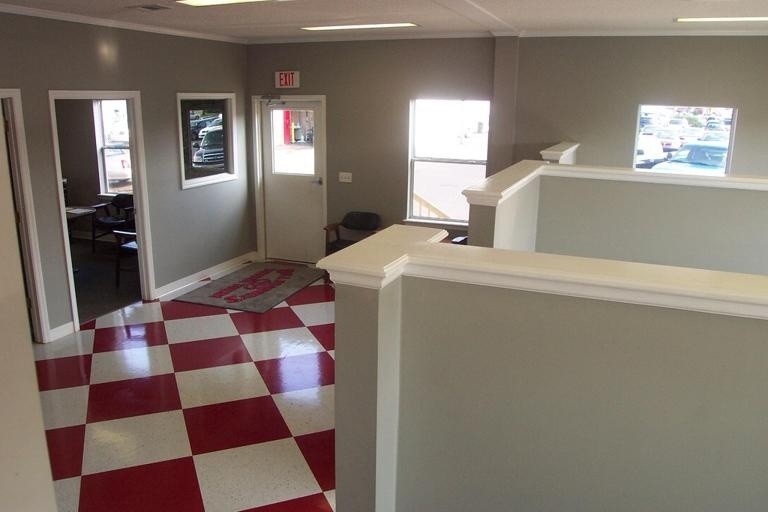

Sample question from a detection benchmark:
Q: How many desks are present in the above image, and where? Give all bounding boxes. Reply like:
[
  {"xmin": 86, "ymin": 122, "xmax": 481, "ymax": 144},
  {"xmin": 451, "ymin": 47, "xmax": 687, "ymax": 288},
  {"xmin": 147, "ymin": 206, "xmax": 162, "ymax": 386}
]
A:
[{"xmin": 66, "ymin": 204, "xmax": 96, "ymax": 255}]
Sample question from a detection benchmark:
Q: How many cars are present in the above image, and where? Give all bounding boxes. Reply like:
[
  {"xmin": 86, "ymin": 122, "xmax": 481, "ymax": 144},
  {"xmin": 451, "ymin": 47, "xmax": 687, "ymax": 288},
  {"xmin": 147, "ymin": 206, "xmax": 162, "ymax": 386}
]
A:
[
  {"xmin": 636, "ymin": 106, "xmax": 732, "ymax": 175},
  {"xmin": 190, "ymin": 114, "xmax": 224, "ymax": 170}
]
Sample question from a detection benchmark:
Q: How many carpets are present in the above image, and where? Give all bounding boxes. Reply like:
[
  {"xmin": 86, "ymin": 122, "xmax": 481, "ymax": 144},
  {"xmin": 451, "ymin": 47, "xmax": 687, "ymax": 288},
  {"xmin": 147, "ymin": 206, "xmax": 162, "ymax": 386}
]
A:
[{"xmin": 172, "ymin": 261, "xmax": 324, "ymax": 314}]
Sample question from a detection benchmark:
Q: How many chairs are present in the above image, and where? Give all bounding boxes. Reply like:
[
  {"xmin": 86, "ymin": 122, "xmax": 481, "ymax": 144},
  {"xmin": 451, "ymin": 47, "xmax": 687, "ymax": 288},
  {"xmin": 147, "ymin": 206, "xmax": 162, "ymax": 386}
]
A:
[
  {"xmin": 93, "ymin": 192, "xmax": 138, "ymax": 287},
  {"xmin": 322, "ymin": 212, "xmax": 382, "ymax": 286}
]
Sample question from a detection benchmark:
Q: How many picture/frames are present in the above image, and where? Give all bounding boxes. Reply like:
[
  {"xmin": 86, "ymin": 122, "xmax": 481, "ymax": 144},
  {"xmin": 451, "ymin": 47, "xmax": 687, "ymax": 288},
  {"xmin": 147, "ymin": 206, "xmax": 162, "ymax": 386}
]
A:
[{"xmin": 175, "ymin": 91, "xmax": 239, "ymax": 191}]
